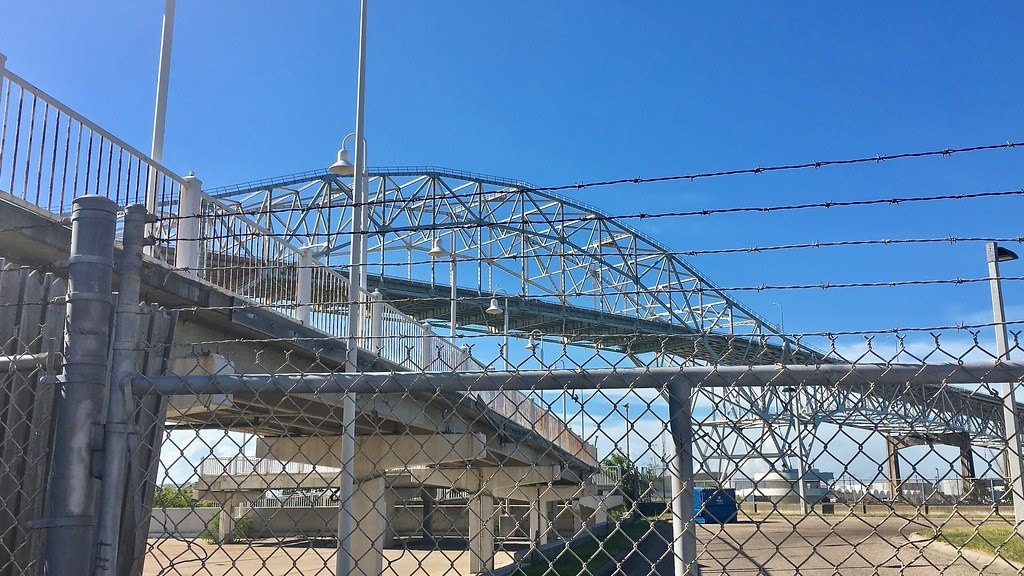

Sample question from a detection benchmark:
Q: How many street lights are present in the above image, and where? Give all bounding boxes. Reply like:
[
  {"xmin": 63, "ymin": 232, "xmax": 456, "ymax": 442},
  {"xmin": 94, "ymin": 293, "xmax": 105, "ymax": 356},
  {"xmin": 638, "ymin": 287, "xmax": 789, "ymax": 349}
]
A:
[
  {"xmin": 485, "ymin": 288, "xmax": 510, "ymax": 415},
  {"xmin": 327, "ymin": 132, "xmax": 367, "ymax": 348},
  {"xmin": 552, "ymin": 359, "xmax": 567, "ymax": 424},
  {"xmin": 427, "ymin": 226, "xmax": 456, "ymax": 373},
  {"xmin": 525, "ymin": 328, "xmax": 544, "ymax": 408},
  {"xmin": 781, "ymin": 387, "xmax": 807, "ymax": 515},
  {"xmin": 985, "ymin": 240, "xmax": 1024, "ymax": 540},
  {"xmin": 773, "ymin": 302, "xmax": 783, "ymax": 332},
  {"xmin": 621, "ymin": 402, "xmax": 629, "ymax": 459}
]
